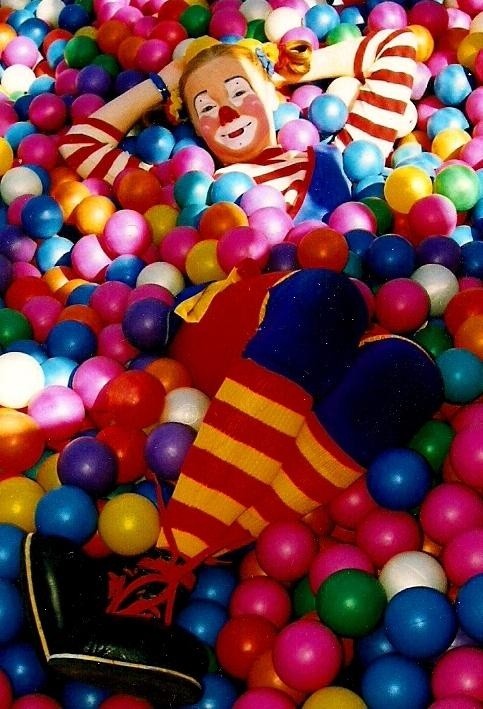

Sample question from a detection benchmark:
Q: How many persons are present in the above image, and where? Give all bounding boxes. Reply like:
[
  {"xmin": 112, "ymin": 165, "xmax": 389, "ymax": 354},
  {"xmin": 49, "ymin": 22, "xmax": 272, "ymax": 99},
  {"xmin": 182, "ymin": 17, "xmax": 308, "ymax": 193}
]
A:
[{"xmin": 22, "ymin": 26, "xmax": 446, "ymax": 707}]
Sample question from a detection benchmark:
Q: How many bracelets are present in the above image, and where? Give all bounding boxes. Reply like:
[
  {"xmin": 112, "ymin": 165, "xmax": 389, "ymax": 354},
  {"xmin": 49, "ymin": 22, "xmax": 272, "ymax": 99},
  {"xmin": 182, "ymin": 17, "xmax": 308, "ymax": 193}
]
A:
[{"xmin": 149, "ymin": 72, "xmax": 171, "ymax": 104}]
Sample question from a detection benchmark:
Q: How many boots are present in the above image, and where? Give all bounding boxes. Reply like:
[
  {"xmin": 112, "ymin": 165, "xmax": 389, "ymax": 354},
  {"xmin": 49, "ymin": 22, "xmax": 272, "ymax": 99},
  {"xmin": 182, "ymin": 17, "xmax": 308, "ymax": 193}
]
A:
[{"xmin": 25, "ymin": 532, "xmax": 208, "ymax": 703}]
